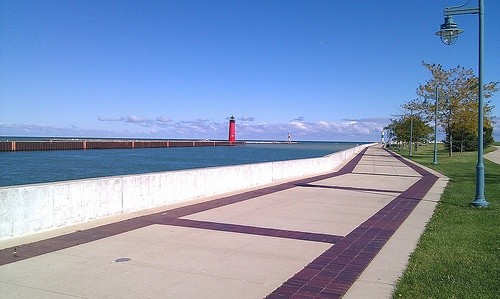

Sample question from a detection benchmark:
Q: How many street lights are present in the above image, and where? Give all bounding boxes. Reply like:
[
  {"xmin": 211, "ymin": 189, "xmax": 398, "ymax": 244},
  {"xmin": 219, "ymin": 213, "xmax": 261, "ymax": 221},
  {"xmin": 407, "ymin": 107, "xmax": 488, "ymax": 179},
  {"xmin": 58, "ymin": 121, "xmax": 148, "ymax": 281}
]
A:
[
  {"xmin": 435, "ymin": 0, "xmax": 492, "ymax": 209},
  {"xmin": 423, "ymin": 84, "xmax": 441, "ymax": 164}
]
